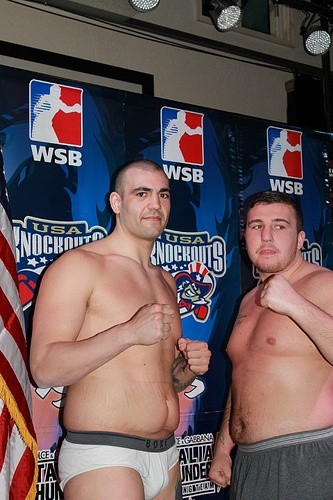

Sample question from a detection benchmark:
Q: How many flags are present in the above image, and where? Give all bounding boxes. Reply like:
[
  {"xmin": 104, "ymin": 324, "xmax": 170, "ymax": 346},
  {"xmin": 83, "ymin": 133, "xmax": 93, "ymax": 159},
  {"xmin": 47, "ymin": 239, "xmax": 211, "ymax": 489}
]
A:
[{"xmin": 0, "ymin": 145, "xmax": 39, "ymax": 500}]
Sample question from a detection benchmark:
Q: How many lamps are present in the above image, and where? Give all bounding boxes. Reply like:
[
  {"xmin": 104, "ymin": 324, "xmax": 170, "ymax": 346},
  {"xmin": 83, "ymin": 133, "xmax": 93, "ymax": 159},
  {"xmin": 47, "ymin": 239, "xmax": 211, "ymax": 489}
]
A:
[
  {"xmin": 208, "ymin": 0, "xmax": 242, "ymax": 32},
  {"xmin": 304, "ymin": 26, "xmax": 332, "ymax": 55},
  {"xmin": 128, "ymin": 0, "xmax": 161, "ymax": 12}
]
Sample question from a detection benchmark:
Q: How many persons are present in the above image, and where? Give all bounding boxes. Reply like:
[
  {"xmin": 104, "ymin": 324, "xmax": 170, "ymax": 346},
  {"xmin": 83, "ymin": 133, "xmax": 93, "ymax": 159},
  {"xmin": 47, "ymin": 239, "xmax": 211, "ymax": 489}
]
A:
[
  {"xmin": 29, "ymin": 157, "xmax": 211, "ymax": 500},
  {"xmin": 209, "ymin": 191, "xmax": 333, "ymax": 500}
]
